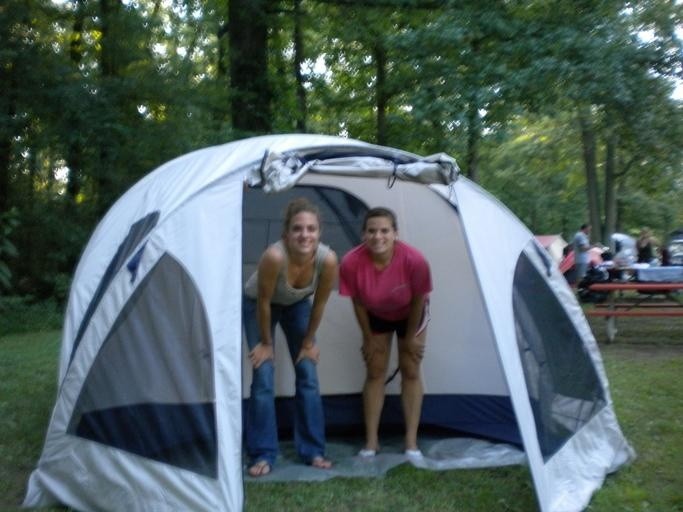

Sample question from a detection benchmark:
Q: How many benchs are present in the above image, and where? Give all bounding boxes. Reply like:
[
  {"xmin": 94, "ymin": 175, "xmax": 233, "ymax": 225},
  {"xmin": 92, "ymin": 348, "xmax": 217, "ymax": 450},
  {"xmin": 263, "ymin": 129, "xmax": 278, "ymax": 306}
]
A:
[{"xmin": 586, "ymin": 261, "xmax": 682, "ymax": 343}]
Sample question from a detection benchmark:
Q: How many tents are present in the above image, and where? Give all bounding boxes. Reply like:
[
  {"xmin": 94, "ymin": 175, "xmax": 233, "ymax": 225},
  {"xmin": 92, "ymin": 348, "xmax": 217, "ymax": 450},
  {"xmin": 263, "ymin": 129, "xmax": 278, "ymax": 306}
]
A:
[{"xmin": 22, "ymin": 134, "xmax": 637, "ymax": 512}]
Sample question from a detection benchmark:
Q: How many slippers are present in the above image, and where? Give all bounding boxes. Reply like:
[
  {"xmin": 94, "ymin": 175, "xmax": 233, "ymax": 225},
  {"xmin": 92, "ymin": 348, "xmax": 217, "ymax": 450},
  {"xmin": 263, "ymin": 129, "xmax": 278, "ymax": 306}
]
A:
[
  {"xmin": 355, "ymin": 448, "xmax": 381, "ymax": 459},
  {"xmin": 313, "ymin": 456, "xmax": 332, "ymax": 469},
  {"xmin": 403, "ymin": 449, "xmax": 424, "ymax": 460},
  {"xmin": 250, "ymin": 462, "xmax": 270, "ymax": 476}
]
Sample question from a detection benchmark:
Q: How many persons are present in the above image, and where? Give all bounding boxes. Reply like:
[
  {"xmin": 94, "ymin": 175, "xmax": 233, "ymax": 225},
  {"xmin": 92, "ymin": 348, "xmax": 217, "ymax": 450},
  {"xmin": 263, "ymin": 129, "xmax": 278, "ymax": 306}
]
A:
[
  {"xmin": 572, "ymin": 223, "xmax": 592, "ymax": 295},
  {"xmin": 575, "ymin": 251, "xmax": 617, "ymax": 300},
  {"xmin": 636, "ymin": 226, "xmax": 651, "ymax": 295},
  {"xmin": 242, "ymin": 197, "xmax": 338, "ymax": 476},
  {"xmin": 337, "ymin": 206, "xmax": 432, "ymax": 464},
  {"xmin": 654, "ymin": 246, "xmax": 673, "ymax": 268}
]
